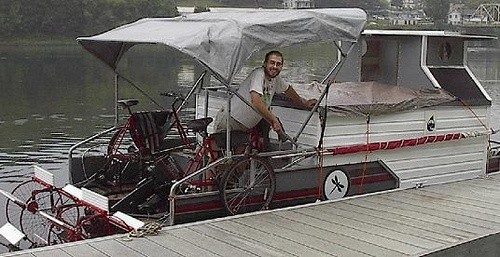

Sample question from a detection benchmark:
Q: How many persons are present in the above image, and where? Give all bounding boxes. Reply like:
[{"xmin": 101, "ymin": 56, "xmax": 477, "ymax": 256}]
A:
[{"xmin": 204, "ymin": 52, "xmax": 320, "ymax": 192}]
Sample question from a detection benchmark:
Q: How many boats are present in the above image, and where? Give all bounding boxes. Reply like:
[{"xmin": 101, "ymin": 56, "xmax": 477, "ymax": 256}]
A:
[{"xmin": 0, "ymin": 6, "xmax": 500, "ymax": 251}]
